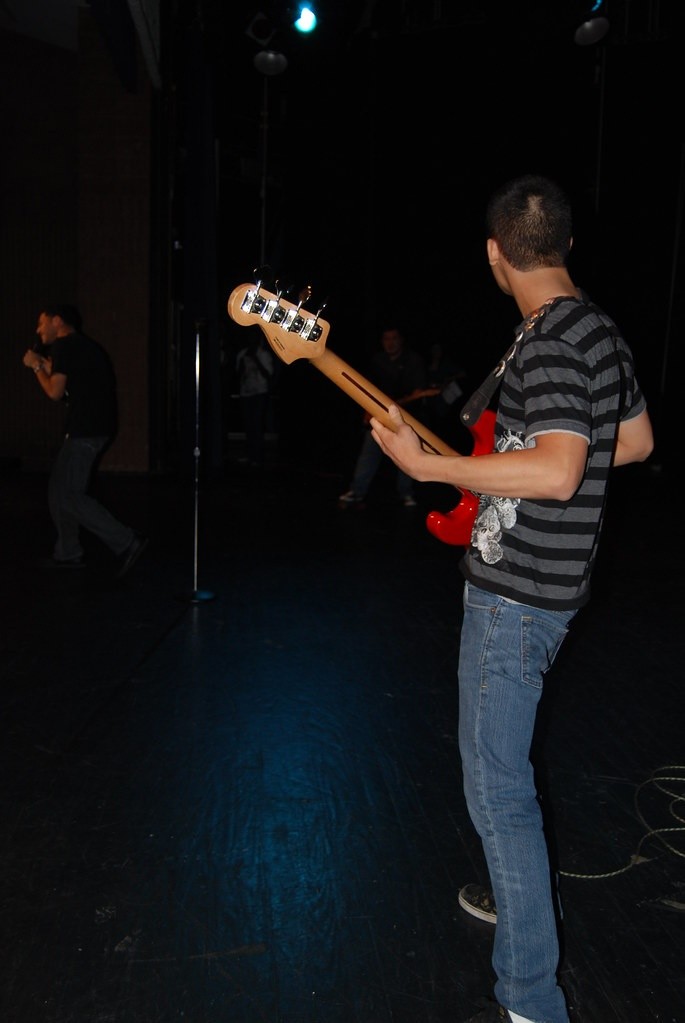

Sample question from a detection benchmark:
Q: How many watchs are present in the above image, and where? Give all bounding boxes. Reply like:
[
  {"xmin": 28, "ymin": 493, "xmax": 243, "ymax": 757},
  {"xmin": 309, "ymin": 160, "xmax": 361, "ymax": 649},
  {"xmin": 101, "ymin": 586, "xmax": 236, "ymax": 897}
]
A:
[{"xmin": 34, "ymin": 365, "xmax": 44, "ymax": 374}]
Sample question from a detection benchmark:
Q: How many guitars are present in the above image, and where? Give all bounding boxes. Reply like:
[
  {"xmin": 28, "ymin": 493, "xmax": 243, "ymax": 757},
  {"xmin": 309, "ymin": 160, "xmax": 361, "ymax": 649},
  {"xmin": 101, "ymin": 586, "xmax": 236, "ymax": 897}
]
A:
[{"xmin": 224, "ymin": 277, "xmax": 498, "ymax": 551}]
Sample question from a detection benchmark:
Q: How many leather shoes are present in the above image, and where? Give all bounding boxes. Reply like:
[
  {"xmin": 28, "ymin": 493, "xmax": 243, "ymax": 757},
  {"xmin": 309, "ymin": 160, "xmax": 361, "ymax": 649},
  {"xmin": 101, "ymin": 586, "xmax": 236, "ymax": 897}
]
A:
[
  {"xmin": 113, "ymin": 530, "xmax": 149, "ymax": 580},
  {"xmin": 36, "ymin": 552, "xmax": 91, "ymax": 569}
]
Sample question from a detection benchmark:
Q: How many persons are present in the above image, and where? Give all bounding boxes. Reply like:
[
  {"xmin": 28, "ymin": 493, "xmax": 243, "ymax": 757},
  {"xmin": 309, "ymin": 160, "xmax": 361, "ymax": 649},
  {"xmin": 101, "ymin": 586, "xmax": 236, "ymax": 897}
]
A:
[
  {"xmin": 236, "ymin": 329, "xmax": 273, "ymax": 465},
  {"xmin": 23, "ymin": 304, "xmax": 149, "ymax": 579},
  {"xmin": 369, "ymin": 175, "xmax": 654, "ymax": 1023},
  {"xmin": 339, "ymin": 327, "xmax": 417, "ymax": 506}
]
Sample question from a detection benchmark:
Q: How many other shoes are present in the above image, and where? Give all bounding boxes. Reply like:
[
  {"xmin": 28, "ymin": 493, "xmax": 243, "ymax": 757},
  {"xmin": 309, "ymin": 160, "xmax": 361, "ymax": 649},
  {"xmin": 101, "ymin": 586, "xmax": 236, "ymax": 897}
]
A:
[
  {"xmin": 402, "ymin": 493, "xmax": 417, "ymax": 506},
  {"xmin": 340, "ymin": 490, "xmax": 365, "ymax": 502},
  {"xmin": 457, "ymin": 883, "xmax": 496, "ymax": 924},
  {"xmin": 464, "ymin": 1006, "xmax": 511, "ymax": 1023}
]
